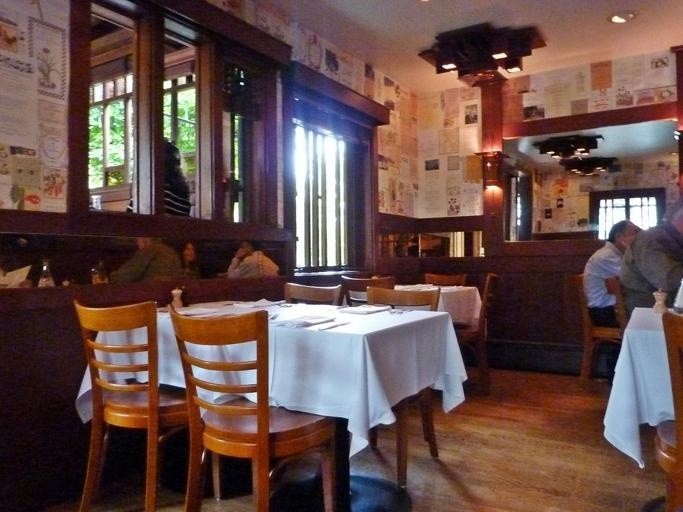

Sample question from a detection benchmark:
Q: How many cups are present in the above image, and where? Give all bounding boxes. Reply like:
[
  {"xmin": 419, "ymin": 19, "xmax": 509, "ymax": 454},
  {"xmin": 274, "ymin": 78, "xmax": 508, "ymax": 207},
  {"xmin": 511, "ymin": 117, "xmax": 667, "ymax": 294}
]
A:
[{"xmin": 90, "ymin": 266, "xmax": 105, "ymax": 284}]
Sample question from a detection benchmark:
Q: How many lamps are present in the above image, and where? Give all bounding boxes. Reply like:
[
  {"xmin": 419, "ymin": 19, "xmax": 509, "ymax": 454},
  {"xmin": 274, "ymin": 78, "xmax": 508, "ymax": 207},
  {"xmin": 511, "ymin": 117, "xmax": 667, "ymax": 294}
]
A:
[
  {"xmin": 416, "ymin": 23, "xmax": 548, "ymax": 88},
  {"xmin": 558, "ymin": 157, "xmax": 618, "ymax": 178},
  {"xmin": 472, "ymin": 150, "xmax": 510, "ymax": 190},
  {"xmin": 530, "ymin": 134, "xmax": 605, "ymax": 160}
]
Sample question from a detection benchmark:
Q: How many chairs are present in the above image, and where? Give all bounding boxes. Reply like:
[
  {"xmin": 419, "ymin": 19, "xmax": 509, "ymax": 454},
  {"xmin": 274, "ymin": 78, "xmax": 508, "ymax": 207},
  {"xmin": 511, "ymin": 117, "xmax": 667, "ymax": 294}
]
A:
[
  {"xmin": 68, "ymin": 300, "xmax": 221, "ymax": 510},
  {"xmin": 572, "ymin": 265, "xmax": 682, "ymax": 510},
  {"xmin": 330, "ymin": 269, "xmax": 507, "ymax": 405},
  {"xmin": 362, "ymin": 280, "xmax": 443, "ymax": 491},
  {"xmin": 283, "ymin": 280, "xmax": 343, "ymax": 306},
  {"xmin": 166, "ymin": 302, "xmax": 348, "ymax": 512}
]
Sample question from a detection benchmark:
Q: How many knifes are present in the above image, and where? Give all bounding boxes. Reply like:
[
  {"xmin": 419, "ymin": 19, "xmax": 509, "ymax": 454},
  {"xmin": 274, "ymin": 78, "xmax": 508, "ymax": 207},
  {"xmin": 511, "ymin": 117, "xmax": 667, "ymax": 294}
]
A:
[{"xmin": 317, "ymin": 320, "xmax": 350, "ymax": 332}]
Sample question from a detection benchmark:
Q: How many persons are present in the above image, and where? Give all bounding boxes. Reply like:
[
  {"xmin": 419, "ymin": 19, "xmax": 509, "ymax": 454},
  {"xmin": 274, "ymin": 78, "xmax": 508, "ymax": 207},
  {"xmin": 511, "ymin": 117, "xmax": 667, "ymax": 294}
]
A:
[
  {"xmin": 178, "ymin": 241, "xmax": 207, "ymax": 278},
  {"xmin": 126, "ymin": 138, "xmax": 191, "ymax": 216},
  {"xmin": 582, "ymin": 219, "xmax": 639, "ymax": 383},
  {"xmin": 107, "ymin": 237, "xmax": 184, "ymax": 282},
  {"xmin": 618, "ymin": 194, "xmax": 683, "ymax": 318},
  {"xmin": 226, "ymin": 240, "xmax": 278, "ymax": 277}
]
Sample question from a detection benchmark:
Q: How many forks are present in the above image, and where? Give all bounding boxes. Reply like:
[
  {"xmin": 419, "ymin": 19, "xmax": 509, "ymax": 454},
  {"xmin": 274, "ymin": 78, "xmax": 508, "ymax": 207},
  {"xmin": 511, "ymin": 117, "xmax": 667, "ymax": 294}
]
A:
[{"xmin": 270, "ymin": 313, "xmax": 281, "ymax": 320}]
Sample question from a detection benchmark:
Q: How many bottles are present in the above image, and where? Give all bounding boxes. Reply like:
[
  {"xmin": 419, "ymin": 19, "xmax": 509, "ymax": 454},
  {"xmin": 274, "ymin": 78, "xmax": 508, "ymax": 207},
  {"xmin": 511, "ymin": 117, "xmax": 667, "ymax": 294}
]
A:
[
  {"xmin": 170, "ymin": 287, "xmax": 183, "ymax": 310},
  {"xmin": 672, "ymin": 277, "xmax": 683, "ymax": 314},
  {"xmin": 181, "ymin": 285, "xmax": 190, "ymax": 309},
  {"xmin": 651, "ymin": 288, "xmax": 670, "ymax": 316},
  {"xmin": 37, "ymin": 259, "xmax": 56, "ymax": 288}
]
